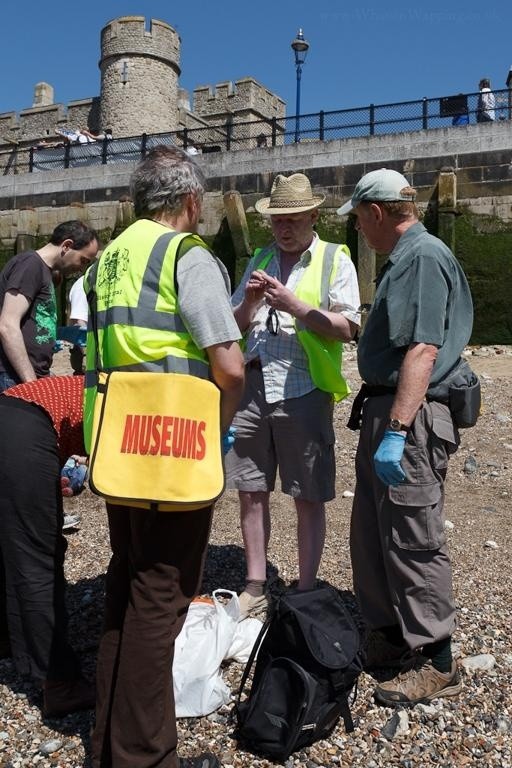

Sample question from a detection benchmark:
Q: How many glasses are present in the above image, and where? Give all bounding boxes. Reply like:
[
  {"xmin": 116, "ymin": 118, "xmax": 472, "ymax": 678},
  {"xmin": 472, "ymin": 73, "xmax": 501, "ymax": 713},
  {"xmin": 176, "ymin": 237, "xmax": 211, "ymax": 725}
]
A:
[{"xmin": 265, "ymin": 305, "xmax": 280, "ymax": 337}]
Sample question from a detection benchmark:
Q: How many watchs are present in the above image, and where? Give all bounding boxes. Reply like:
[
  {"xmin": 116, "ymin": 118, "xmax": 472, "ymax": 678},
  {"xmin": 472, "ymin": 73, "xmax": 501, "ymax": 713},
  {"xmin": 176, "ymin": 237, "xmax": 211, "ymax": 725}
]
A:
[{"xmin": 386, "ymin": 417, "xmax": 411, "ymax": 433}]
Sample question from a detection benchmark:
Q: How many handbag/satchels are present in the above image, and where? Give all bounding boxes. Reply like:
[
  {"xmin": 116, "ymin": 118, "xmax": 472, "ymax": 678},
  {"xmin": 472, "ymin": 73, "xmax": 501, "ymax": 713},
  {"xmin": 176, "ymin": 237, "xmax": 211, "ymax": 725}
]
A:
[
  {"xmin": 448, "ymin": 367, "xmax": 481, "ymax": 428},
  {"xmin": 83, "ymin": 366, "xmax": 226, "ymax": 514},
  {"xmin": 172, "ymin": 587, "xmax": 250, "ymax": 723}
]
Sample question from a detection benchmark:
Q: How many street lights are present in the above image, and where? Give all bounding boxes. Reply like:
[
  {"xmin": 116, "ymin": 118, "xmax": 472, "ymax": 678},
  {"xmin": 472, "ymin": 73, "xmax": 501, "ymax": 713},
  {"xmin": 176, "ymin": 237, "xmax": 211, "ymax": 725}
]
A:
[{"xmin": 291, "ymin": 27, "xmax": 309, "ymax": 144}]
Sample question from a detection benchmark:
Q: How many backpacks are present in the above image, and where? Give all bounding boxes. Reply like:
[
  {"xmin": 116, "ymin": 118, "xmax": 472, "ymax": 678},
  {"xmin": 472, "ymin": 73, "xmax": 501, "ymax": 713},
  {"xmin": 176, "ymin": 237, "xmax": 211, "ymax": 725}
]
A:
[{"xmin": 231, "ymin": 583, "xmax": 366, "ymax": 764}]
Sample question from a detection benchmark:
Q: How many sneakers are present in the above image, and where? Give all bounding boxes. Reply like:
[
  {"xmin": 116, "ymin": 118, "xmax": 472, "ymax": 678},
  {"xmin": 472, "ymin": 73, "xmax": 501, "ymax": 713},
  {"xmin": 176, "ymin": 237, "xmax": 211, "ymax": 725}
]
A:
[
  {"xmin": 373, "ymin": 654, "xmax": 463, "ymax": 708},
  {"xmin": 42, "ymin": 675, "xmax": 98, "ymax": 719},
  {"xmin": 176, "ymin": 752, "xmax": 222, "ymax": 768},
  {"xmin": 358, "ymin": 628, "xmax": 410, "ymax": 674},
  {"xmin": 227, "ymin": 590, "xmax": 270, "ymax": 625}
]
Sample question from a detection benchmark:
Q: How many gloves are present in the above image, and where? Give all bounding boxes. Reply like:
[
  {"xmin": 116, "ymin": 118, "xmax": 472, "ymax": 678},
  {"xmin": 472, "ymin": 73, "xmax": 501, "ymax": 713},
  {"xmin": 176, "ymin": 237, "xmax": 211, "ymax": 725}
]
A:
[
  {"xmin": 222, "ymin": 426, "xmax": 237, "ymax": 456},
  {"xmin": 373, "ymin": 426, "xmax": 409, "ymax": 486}
]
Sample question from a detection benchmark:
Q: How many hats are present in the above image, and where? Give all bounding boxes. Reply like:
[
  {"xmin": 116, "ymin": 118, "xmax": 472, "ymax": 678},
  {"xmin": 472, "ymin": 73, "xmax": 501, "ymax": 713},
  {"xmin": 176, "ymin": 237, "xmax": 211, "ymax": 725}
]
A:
[
  {"xmin": 254, "ymin": 172, "xmax": 326, "ymax": 215},
  {"xmin": 335, "ymin": 167, "xmax": 417, "ymax": 216}
]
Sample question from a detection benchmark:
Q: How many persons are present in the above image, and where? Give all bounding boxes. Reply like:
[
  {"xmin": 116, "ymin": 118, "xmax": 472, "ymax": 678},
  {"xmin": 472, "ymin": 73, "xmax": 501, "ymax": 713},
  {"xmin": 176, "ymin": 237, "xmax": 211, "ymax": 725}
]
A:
[
  {"xmin": 336, "ymin": 168, "xmax": 481, "ymax": 707},
  {"xmin": 54, "ymin": 126, "xmax": 113, "ymax": 143},
  {"xmin": 0, "ymin": 219, "xmax": 99, "ymax": 715},
  {"xmin": 83, "ymin": 144, "xmax": 246, "ymax": 768},
  {"xmin": 452, "ymin": 78, "xmax": 496, "ymax": 125},
  {"xmin": 232, "ymin": 173, "xmax": 361, "ymax": 613}
]
